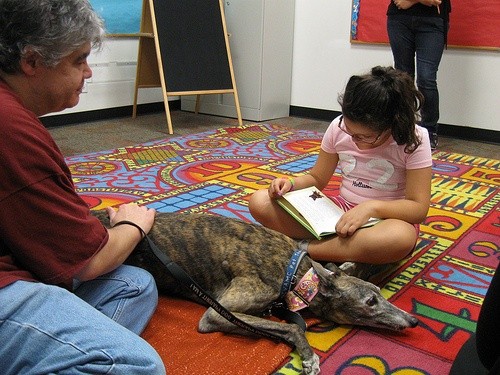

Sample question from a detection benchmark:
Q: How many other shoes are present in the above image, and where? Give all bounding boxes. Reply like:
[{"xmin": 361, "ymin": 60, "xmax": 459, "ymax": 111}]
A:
[
  {"xmin": 338, "ymin": 261, "xmax": 374, "ymax": 278},
  {"xmin": 428, "ymin": 133, "xmax": 439, "ymax": 149}
]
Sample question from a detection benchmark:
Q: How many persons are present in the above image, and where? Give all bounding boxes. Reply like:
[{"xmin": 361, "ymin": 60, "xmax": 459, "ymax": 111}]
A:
[
  {"xmin": 387, "ymin": 0, "xmax": 452, "ymax": 150},
  {"xmin": 0, "ymin": 0, "xmax": 166, "ymax": 375},
  {"xmin": 249, "ymin": 66, "xmax": 432, "ymax": 263}
]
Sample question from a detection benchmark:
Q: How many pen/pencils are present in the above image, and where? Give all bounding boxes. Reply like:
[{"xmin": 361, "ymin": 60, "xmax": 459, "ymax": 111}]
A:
[{"xmin": 437, "ymin": 5, "xmax": 442, "ymax": 15}]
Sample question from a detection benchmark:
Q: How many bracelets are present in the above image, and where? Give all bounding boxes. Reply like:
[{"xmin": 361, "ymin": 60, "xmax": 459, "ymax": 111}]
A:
[{"xmin": 287, "ymin": 177, "xmax": 294, "ymax": 191}]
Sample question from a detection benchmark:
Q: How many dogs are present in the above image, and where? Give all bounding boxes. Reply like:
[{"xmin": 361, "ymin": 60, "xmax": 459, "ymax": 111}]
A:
[{"xmin": 88, "ymin": 207, "xmax": 421, "ymax": 375}]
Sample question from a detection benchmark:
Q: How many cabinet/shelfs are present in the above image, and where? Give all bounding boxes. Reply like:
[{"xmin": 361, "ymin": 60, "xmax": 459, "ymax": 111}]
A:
[{"xmin": 181, "ymin": 0, "xmax": 297, "ymax": 122}]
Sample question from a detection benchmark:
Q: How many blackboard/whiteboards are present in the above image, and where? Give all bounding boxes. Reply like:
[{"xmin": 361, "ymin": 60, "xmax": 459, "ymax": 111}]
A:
[{"xmin": 128, "ymin": 0, "xmax": 246, "ymax": 137}]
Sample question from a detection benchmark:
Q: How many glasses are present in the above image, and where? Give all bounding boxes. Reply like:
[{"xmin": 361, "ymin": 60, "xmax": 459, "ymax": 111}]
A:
[{"xmin": 337, "ymin": 116, "xmax": 382, "ymax": 147}]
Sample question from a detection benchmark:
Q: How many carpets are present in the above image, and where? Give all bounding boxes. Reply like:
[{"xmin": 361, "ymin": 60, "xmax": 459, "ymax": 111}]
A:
[{"xmin": 65, "ymin": 123, "xmax": 499, "ymax": 375}]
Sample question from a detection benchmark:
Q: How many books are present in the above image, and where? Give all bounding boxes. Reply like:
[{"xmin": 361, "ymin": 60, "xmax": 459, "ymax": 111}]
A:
[{"xmin": 277, "ymin": 185, "xmax": 380, "ymax": 241}]
